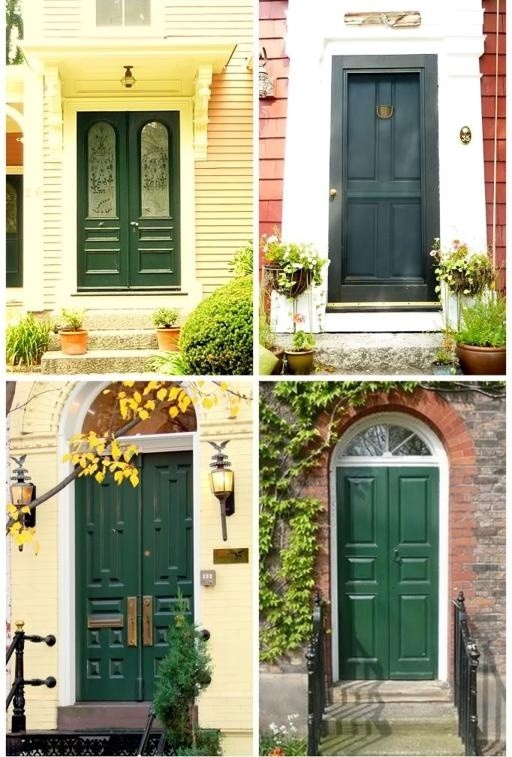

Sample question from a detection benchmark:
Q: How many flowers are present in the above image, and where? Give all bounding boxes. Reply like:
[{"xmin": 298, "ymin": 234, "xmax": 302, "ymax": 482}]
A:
[
  {"xmin": 430, "ymin": 238, "xmax": 485, "ymax": 274},
  {"xmin": 262, "ymin": 240, "xmax": 330, "ymax": 271}
]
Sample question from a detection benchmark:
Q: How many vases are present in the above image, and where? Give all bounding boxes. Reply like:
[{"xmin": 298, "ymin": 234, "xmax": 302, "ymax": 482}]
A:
[
  {"xmin": 269, "ymin": 271, "xmax": 318, "ymax": 296},
  {"xmin": 446, "ymin": 272, "xmax": 477, "ymax": 292}
]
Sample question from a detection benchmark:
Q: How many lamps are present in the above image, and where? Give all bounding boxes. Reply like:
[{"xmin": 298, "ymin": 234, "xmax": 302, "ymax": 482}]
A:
[
  {"xmin": 210, "ymin": 438, "xmax": 234, "ymax": 542},
  {"xmin": 7, "ymin": 453, "xmax": 38, "ymax": 555},
  {"xmin": 122, "ymin": 66, "xmax": 138, "ymax": 87}
]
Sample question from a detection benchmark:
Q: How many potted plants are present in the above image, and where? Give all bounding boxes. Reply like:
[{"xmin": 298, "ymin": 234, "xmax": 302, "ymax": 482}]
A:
[
  {"xmin": 286, "ymin": 330, "xmax": 318, "ymax": 370},
  {"xmin": 457, "ymin": 293, "xmax": 507, "ymax": 376},
  {"xmin": 152, "ymin": 305, "xmax": 182, "ymax": 349},
  {"xmin": 59, "ymin": 306, "xmax": 89, "ymax": 355}
]
